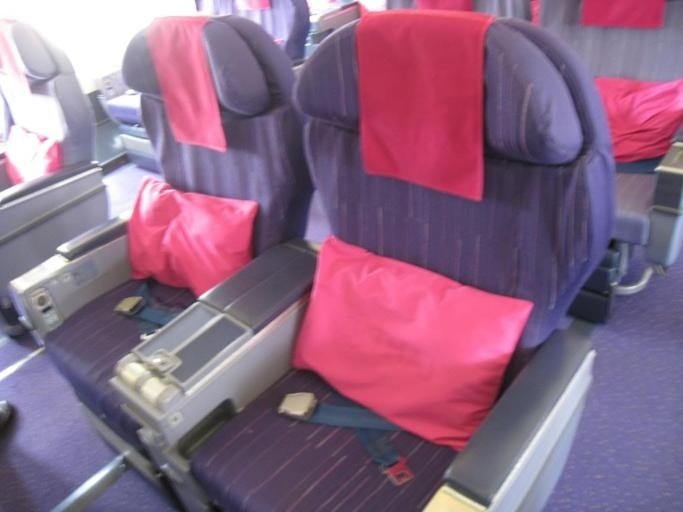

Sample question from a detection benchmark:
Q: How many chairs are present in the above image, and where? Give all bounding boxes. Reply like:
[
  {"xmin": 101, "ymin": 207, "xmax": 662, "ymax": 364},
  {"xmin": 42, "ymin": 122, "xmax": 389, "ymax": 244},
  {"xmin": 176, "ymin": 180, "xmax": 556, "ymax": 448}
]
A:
[
  {"xmin": 103, "ymin": 2, "xmax": 307, "ymax": 171},
  {"xmin": 1, "ymin": 11, "xmax": 110, "ymax": 337},
  {"xmin": 311, "ymin": 2, "xmax": 530, "ymax": 38},
  {"xmin": 510, "ymin": 1, "xmax": 682, "ymax": 293},
  {"xmin": 138, "ymin": 6, "xmax": 616, "ymax": 512},
  {"xmin": 6, "ymin": 14, "xmax": 313, "ymax": 493}
]
